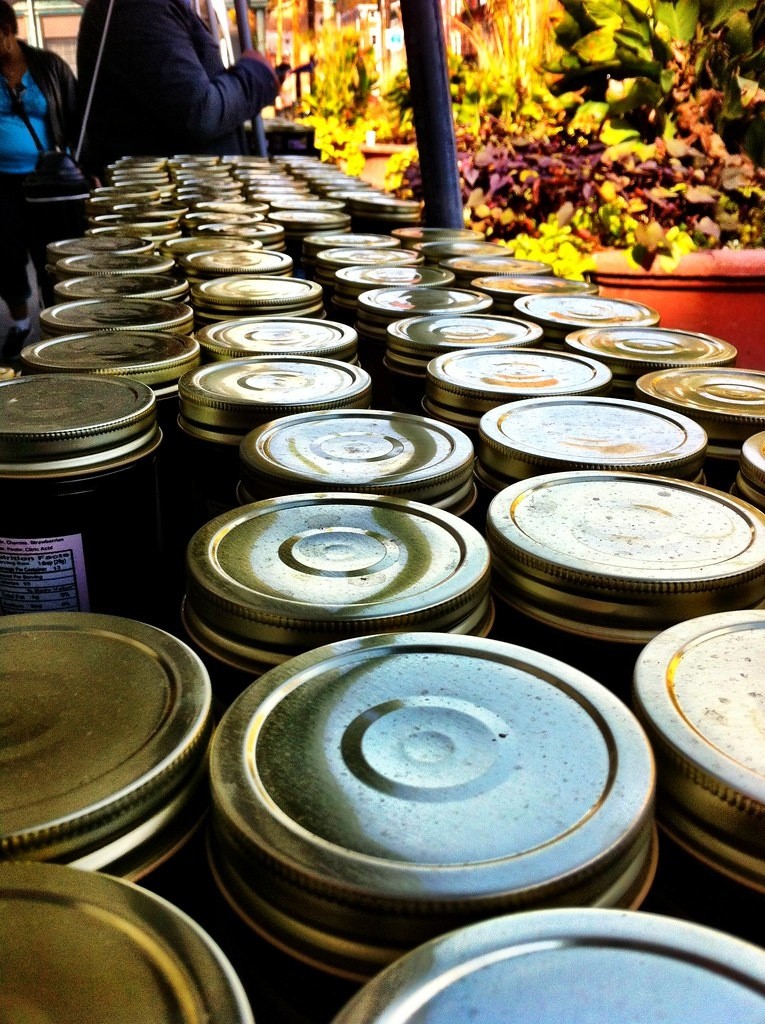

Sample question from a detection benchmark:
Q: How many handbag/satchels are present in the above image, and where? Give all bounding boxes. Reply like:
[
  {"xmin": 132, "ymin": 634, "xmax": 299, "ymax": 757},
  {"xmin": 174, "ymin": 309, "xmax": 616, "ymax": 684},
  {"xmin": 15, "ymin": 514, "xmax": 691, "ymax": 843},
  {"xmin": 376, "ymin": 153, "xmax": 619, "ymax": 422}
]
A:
[{"xmin": 24, "ymin": 153, "xmax": 91, "ymax": 232}]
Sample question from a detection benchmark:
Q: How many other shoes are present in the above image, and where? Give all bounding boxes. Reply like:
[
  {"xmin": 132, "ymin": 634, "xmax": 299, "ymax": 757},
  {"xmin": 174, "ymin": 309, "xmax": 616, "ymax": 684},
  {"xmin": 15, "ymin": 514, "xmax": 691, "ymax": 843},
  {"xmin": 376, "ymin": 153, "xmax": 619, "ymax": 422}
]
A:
[{"xmin": 0, "ymin": 325, "xmax": 34, "ymax": 362}]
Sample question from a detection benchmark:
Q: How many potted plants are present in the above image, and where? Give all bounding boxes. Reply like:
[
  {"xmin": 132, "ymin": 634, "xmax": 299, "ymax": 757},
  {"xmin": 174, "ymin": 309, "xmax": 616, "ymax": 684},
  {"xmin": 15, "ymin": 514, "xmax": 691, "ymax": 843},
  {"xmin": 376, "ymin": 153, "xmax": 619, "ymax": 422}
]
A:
[
  {"xmin": 357, "ymin": 68, "xmax": 416, "ymax": 187},
  {"xmin": 541, "ymin": 0, "xmax": 765, "ymax": 372}
]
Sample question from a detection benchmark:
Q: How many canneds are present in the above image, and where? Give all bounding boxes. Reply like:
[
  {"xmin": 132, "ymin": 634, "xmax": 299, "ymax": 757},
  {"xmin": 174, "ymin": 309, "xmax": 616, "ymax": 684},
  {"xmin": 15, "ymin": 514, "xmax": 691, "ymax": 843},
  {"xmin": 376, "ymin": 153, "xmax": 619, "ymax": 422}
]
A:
[{"xmin": 1, "ymin": 156, "xmax": 764, "ymax": 1024}]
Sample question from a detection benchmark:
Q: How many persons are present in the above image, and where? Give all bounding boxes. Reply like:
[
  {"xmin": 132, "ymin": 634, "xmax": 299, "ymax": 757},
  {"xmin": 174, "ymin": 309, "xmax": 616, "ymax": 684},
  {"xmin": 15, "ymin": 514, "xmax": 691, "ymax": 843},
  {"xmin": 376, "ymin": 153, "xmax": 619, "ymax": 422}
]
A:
[
  {"xmin": 75, "ymin": 1, "xmax": 282, "ymax": 188},
  {"xmin": 0, "ymin": 1, "xmax": 87, "ymax": 358}
]
